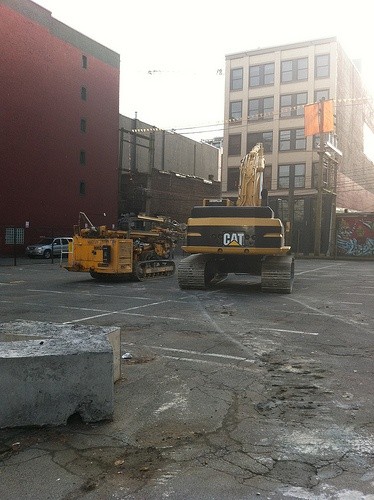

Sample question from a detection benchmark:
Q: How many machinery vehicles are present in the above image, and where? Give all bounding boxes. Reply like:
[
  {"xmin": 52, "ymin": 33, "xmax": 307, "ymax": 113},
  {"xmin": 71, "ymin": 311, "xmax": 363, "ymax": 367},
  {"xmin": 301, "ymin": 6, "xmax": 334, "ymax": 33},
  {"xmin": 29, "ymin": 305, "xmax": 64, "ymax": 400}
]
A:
[
  {"xmin": 176, "ymin": 141, "xmax": 294, "ymax": 294},
  {"xmin": 65, "ymin": 211, "xmax": 185, "ymax": 281}
]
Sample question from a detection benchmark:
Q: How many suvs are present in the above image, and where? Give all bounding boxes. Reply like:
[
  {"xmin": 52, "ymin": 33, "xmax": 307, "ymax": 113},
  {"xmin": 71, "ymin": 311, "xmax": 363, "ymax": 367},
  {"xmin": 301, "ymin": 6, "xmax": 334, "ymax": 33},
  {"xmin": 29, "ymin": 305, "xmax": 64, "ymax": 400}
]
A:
[{"xmin": 25, "ymin": 237, "xmax": 74, "ymax": 258}]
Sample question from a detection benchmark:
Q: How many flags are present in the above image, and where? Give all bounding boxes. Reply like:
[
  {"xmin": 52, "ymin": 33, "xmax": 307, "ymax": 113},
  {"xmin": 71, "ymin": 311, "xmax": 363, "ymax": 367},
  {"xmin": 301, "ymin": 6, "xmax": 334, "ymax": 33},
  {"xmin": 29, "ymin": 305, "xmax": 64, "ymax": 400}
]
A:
[{"xmin": 303, "ymin": 99, "xmax": 335, "ymax": 138}]
